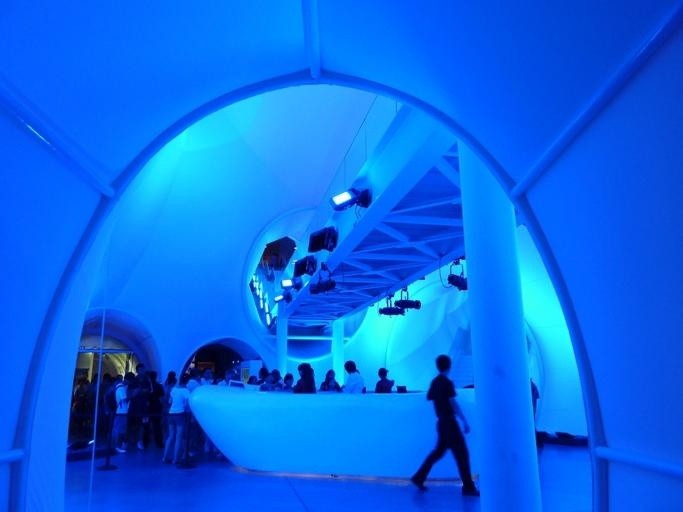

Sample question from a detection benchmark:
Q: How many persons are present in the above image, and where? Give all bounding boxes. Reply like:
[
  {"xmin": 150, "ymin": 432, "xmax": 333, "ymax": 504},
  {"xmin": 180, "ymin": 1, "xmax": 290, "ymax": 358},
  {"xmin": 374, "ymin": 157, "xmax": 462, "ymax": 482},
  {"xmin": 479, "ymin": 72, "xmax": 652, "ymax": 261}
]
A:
[
  {"xmin": 341, "ymin": 361, "xmax": 366, "ymax": 394},
  {"xmin": 291, "ymin": 363, "xmax": 316, "ymax": 393},
  {"xmin": 409, "ymin": 355, "xmax": 480, "ymax": 496},
  {"xmin": 69, "ymin": 363, "xmax": 240, "ymax": 467},
  {"xmin": 248, "ymin": 368, "xmax": 294, "ymax": 392},
  {"xmin": 318, "ymin": 370, "xmax": 341, "ymax": 393},
  {"xmin": 375, "ymin": 368, "xmax": 394, "ymax": 392}
]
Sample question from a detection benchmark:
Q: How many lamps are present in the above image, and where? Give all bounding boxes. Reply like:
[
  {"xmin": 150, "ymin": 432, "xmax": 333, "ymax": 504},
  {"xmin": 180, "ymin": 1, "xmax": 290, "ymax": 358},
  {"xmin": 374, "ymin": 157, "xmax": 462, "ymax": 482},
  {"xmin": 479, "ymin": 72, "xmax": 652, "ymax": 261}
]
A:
[
  {"xmin": 380, "ymin": 253, "xmax": 468, "ymax": 318},
  {"xmin": 273, "ymin": 184, "xmax": 372, "ymax": 304}
]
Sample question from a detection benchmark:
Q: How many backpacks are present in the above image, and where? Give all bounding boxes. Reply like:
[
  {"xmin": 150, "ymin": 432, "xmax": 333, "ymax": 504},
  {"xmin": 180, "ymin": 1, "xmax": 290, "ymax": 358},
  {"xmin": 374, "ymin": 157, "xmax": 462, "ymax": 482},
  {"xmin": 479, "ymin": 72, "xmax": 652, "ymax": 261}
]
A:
[{"xmin": 103, "ymin": 383, "xmax": 125, "ymax": 415}]
